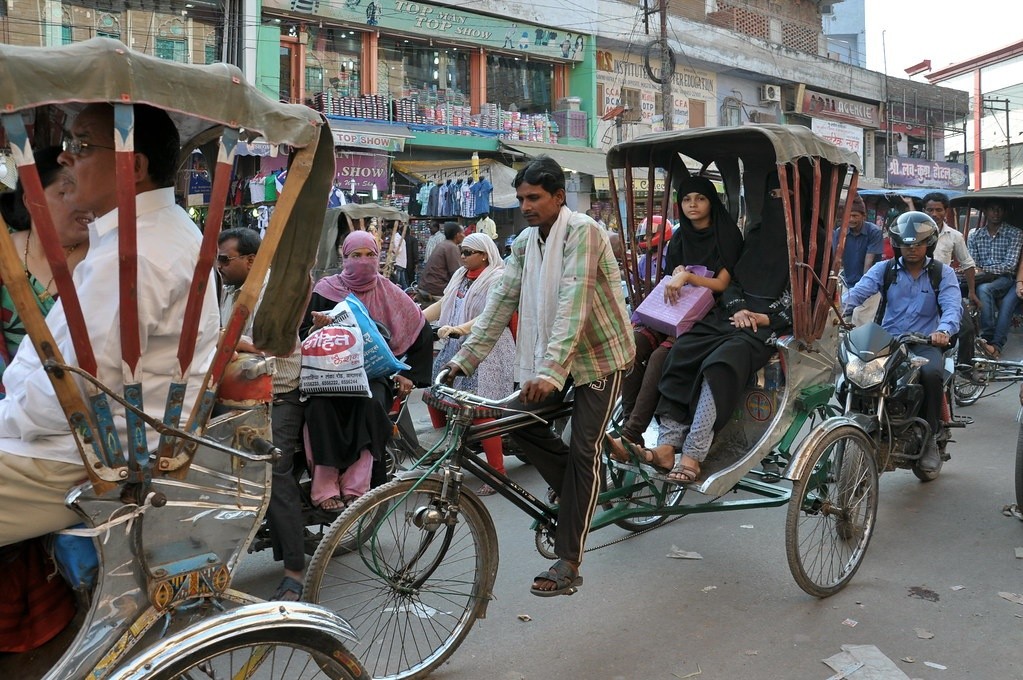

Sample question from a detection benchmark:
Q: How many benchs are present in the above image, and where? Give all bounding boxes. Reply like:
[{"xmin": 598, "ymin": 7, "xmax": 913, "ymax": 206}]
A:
[
  {"xmin": 749, "ymin": 303, "xmax": 844, "ymax": 389},
  {"xmin": 52, "ymin": 355, "xmax": 279, "ymax": 590}
]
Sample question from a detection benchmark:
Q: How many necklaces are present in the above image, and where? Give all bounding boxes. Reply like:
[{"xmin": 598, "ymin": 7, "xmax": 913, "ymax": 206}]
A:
[{"xmin": 25, "ymin": 227, "xmax": 80, "ymax": 302}]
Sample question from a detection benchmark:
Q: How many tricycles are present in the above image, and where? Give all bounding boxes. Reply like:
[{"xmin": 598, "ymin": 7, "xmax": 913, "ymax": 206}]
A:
[
  {"xmin": 950, "ymin": 184, "xmax": 1023, "ymax": 407},
  {"xmin": 295, "ymin": 120, "xmax": 883, "ymax": 680},
  {"xmin": 0, "ymin": 32, "xmax": 377, "ymax": 680}
]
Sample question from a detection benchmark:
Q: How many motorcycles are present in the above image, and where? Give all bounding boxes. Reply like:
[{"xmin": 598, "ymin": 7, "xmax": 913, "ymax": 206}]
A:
[{"xmin": 833, "ymin": 270, "xmax": 975, "ymax": 541}]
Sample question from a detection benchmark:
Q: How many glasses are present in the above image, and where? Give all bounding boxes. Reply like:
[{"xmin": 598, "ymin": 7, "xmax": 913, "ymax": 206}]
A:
[
  {"xmin": 460, "ymin": 249, "xmax": 481, "ymax": 256},
  {"xmin": 218, "ymin": 254, "xmax": 245, "ymax": 265},
  {"xmin": 63, "ymin": 137, "xmax": 115, "ymax": 155}
]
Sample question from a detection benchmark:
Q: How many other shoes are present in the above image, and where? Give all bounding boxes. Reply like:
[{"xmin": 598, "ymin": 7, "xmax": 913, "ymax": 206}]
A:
[
  {"xmin": 606, "ymin": 432, "xmax": 630, "ymax": 460},
  {"xmin": 919, "ymin": 436, "xmax": 940, "ymax": 471},
  {"xmin": 610, "ymin": 452, "xmax": 618, "ymax": 460}
]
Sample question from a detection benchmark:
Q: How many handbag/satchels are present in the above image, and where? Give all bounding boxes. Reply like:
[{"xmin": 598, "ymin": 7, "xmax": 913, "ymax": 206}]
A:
[
  {"xmin": 298, "ymin": 303, "xmax": 373, "ymax": 397},
  {"xmin": 630, "ymin": 264, "xmax": 716, "ymax": 339},
  {"xmin": 338, "ymin": 292, "xmax": 412, "ymax": 378}
]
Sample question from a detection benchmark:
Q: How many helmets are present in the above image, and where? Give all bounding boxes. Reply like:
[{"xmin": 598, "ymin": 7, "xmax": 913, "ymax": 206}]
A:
[
  {"xmin": 504, "ymin": 234, "xmax": 516, "ymax": 247},
  {"xmin": 888, "ymin": 211, "xmax": 940, "ymax": 260},
  {"xmin": 636, "ymin": 215, "xmax": 673, "ymax": 248}
]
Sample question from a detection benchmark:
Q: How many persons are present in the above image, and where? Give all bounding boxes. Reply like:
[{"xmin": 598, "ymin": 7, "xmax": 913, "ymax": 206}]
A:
[
  {"xmin": 417, "ymin": 220, "xmax": 464, "ymax": 302},
  {"xmin": 628, "ymin": 159, "xmax": 838, "ymax": 483},
  {"xmin": 446, "ymin": 155, "xmax": 639, "ymax": 597},
  {"xmin": 216, "ymin": 227, "xmax": 305, "ymax": 606},
  {"xmin": 604, "ymin": 175, "xmax": 744, "ymax": 466},
  {"xmin": 627, "ymin": 214, "xmax": 674, "ymax": 312},
  {"xmin": 298, "ymin": 231, "xmax": 434, "ymax": 512},
  {"xmin": 1, "ymin": 103, "xmax": 221, "ymax": 549},
  {"xmin": 420, "ymin": 233, "xmax": 518, "ymax": 495},
  {"xmin": 423, "ymin": 221, "xmax": 446, "ymax": 267},
  {"xmin": 474, "ymin": 212, "xmax": 499, "ymax": 241},
  {"xmin": 842, "ymin": 210, "xmax": 963, "ymax": 470},
  {"xmin": 831, "ymin": 188, "xmax": 1022, "ymax": 383},
  {"xmin": 1, "ymin": 147, "xmax": 91, "ymax": 363},
  {"xmin": 378, "ymin": 223, "xmax": 420, "ymax": 291}
]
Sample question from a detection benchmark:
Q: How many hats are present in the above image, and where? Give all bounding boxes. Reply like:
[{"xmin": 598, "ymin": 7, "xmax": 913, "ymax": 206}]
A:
[{"xmin": 845, "ymin": 190, "xmax": 866, "ymax": 214}]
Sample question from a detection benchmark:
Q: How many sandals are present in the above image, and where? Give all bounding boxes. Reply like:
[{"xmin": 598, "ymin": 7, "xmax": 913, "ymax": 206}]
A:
[{"xmin": 974, "ymin": 338, "xmax": 999, "ymax": 361}]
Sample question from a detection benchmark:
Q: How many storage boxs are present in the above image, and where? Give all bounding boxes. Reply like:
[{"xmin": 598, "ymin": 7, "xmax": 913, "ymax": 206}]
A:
[
  {"xmin": 315, "ymin": 71, "xmax": 559, "ymax": 144},
  {"xmin": 636, "ymin": 274, "xmax": 715, "ymax": 339}
]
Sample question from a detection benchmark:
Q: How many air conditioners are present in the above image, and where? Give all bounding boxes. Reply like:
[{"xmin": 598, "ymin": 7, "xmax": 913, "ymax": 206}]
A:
[{"xmin": 761, "ymin": 84, "xmax": 781, "ymax": 102}]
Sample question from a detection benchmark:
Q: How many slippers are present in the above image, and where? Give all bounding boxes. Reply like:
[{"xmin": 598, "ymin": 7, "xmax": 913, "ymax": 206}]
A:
[
  {"xmin": 630, "ymin": 443, "xmax": 670, "ymax": 474},
  {"xmin": 320, "ymin": 495, "xmax": 347, "ymax": 512},
  {"xmin": 530, "ymin": 560, "xmax": 584, "ymax": 597},
  {"xmin": 267, "ymin": 574, "xmax": 302, "ymax": 603},
  {"xmin": 341, "ymin": 495, "xmax": 356, "ymax": 507},
  {"xmin": 666, "ymin": 463, "xmax": 699, "ymax": 483},
  {"xmin": 474, "ymin": 478, "xmax": 511, "ymax": 495}
]
